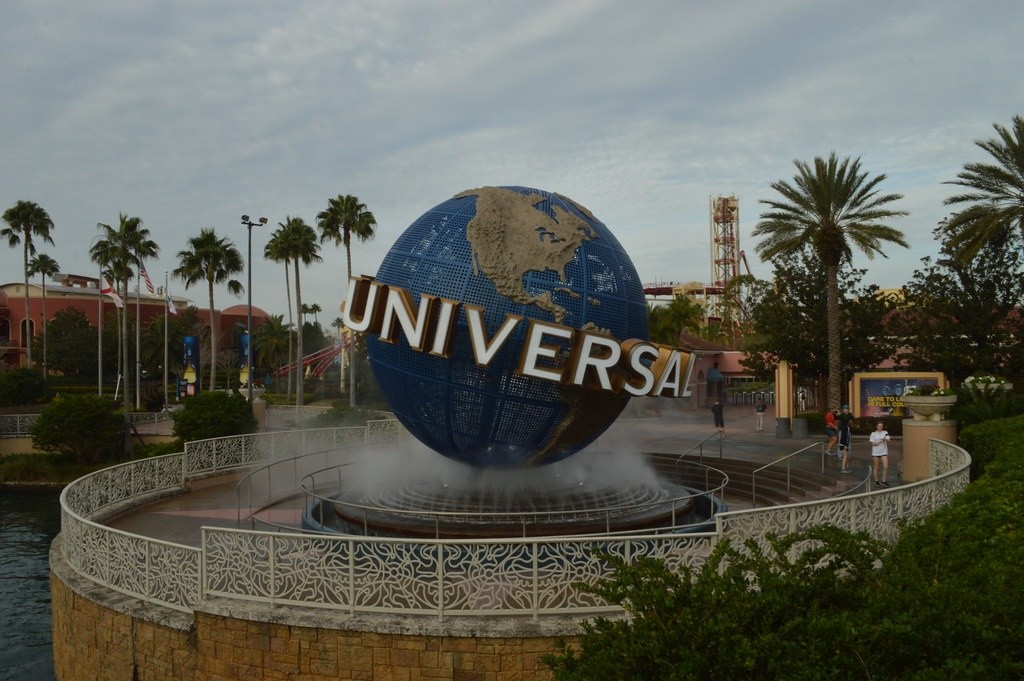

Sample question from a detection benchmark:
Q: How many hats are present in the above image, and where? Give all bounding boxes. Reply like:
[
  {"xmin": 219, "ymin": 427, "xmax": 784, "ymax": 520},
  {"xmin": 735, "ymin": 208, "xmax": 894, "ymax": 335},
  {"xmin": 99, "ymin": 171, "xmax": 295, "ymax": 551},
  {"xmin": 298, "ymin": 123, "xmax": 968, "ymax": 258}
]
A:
[{"xmin": 843, "ymin": 404, "xmax": 850, "ymax": 409}]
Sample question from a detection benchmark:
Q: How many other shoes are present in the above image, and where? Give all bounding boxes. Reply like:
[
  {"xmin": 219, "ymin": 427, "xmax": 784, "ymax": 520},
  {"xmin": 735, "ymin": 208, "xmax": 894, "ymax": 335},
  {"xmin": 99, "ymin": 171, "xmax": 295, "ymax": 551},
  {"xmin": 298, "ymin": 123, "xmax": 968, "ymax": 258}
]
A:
[
  {"xmin": 847, "ymin": 469, "xmax": 852, "ymax": 472},
  {"xmin": 876, "ymin": 480, "xmax": 881, "ymax": 486},
  {"xmin": 841, "ymin": 470, "xmax": 849, "ymax": 473},
  {"xmin": 825, "ymin": 450, "xmax": 831, "ymax": 454},
  {"xmin": 881, "ymin": 480, "xmax": 889, "ymax": 487}
]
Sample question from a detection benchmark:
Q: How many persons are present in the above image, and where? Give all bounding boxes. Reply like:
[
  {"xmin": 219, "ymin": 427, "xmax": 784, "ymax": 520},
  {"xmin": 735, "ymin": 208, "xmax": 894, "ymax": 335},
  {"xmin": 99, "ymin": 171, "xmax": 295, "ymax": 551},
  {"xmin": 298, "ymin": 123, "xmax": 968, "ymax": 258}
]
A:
[
  {"xmin": 711, "ymin": 399, "xmax": 727, "ymax": 440},
  {"xmin": 836, "ymin": 404, "xmax": 854, "ymax": 461},
  {"xmin": 825, "ymin": 406, "xmax": 838, "ymax": 456},
  {"xmin": 754, "ymin": 398, "xmax": 767, "ymax": 431},
  {"xmin": 799, "ymin": 389, "xmax": 806, "ymax": 412},
  {"xmin": 840, "ymin": 416, "xmax": 853, "ymax": 475},
  {"xmin": 869, "ymin": 420, "xmax": 891, "ymax": 488}
]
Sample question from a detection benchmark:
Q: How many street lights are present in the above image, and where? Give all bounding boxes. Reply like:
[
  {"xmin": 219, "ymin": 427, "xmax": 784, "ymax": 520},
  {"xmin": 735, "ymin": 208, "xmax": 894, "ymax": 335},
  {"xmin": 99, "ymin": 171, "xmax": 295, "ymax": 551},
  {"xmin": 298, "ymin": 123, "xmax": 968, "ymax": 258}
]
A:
[{"xmin": 241, "ymin": 214, "xmax": 268, "ymax": 410}]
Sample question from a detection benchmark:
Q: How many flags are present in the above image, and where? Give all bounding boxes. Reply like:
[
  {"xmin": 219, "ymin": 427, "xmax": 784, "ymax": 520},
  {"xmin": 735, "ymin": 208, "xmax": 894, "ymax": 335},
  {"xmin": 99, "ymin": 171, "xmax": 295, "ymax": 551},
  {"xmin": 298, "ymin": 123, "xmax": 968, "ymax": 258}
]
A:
[
  {"xmin": 140, "ymin": 259, "xmax": 154, "ymax": 293},
  {"xmin": 101, "ymin": 275, "xmax": 126, "ymax": 308},
  {"xmin": 167, "ymin": 289, "xmax": 177, "ymax": 315}
]
